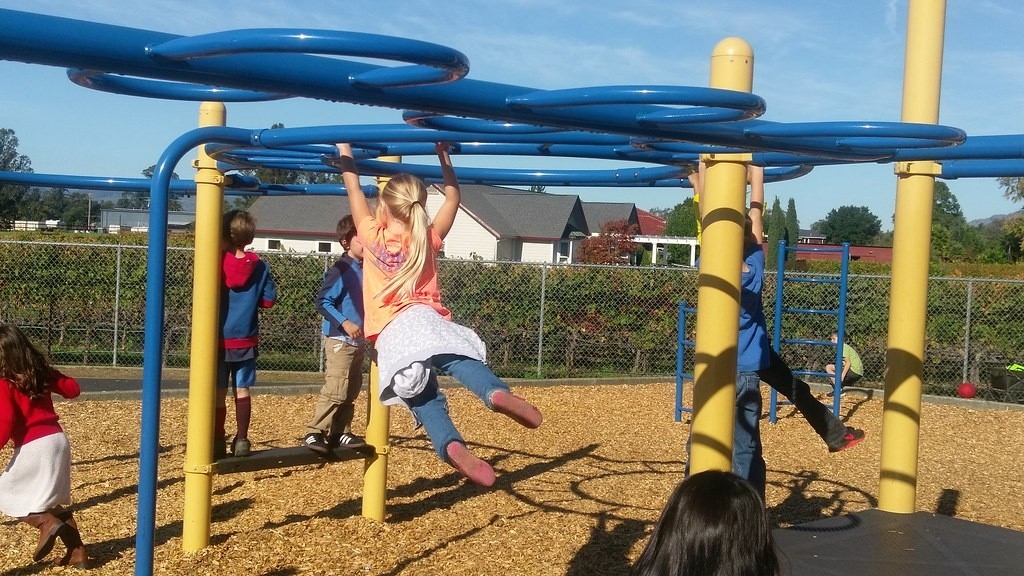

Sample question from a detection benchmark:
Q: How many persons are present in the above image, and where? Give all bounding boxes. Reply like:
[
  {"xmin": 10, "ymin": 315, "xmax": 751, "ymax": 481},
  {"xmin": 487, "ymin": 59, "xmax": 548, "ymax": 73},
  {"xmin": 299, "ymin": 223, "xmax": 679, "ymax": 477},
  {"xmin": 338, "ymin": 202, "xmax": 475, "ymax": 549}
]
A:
[
  {"xmin": 213, "ymin": 208, "xmax": 277, "ymax": 456},
  {"xmin": 1, "ymin": 323, "xmax": 91, "ymax": 570},
  {"xmin": 301, "ymin": 215, "xmax": 366, "ymax": 454},
  {"xmin": 825, "ymin": 329, "xmax": 864, "ymax": 397},
  {"xmin": 686, "ymin": 160, "xmax": 865, "ymax": 511},
  {"xmin": 333, "ymin": 139, "xmax": 542, "ymax": 488},
  {"xmin": 629, "ymin": 470, "xmax": 781, "ymax": 576}
]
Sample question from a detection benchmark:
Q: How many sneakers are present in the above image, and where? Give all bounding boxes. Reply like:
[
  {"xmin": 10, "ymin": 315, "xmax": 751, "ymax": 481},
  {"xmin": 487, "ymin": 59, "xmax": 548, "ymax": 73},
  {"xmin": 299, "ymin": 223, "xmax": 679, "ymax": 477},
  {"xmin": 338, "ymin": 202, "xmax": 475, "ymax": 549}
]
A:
[
  {"xmin": 304, "ymin": 433, "xmax": 328, "ymax": 455},
  {"xmin": 446, "ymin": 441, "xmax": 496, "ymax": 488},
  {"xmin": 212, "ymin": 439, "xmax": 227, "ymax": 457},
  {"xmin": 491, "ymin": 390, "xmax": 543, "ymax": 429},
  {"xmin": 829, "ymin": 426, "xmax": 866, "ymax": 452},
  {"xmin": 230, "ymin": 437, "xmax": 251, "ymax": 457},
  {"xmin": 327, "ymin": 433, "xmax": 365, "ymax": 449},
  {"xmin": 825, "ymin": 388, "xmax": 846, "ymax": 398}
]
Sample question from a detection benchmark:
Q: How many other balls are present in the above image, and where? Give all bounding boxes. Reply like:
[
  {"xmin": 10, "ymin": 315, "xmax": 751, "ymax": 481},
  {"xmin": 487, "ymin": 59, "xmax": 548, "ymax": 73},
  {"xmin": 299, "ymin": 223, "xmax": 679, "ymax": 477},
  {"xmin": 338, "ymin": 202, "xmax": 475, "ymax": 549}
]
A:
[{"xmin": 957, "ymin": 382, "xmax": 977, "ymax": 399}]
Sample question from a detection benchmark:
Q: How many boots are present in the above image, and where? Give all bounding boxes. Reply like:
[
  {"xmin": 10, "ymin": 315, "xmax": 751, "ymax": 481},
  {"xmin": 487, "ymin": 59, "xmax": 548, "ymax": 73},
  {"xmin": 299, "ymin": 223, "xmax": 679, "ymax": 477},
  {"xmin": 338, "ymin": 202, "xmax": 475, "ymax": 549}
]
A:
[
  {"xmin": 55, "ymin": 512, "xmax": 89, "ymax": 569},
  {"xmin": 17, "ymin": 512, "xmax": 65, "ymax": 561}
]
